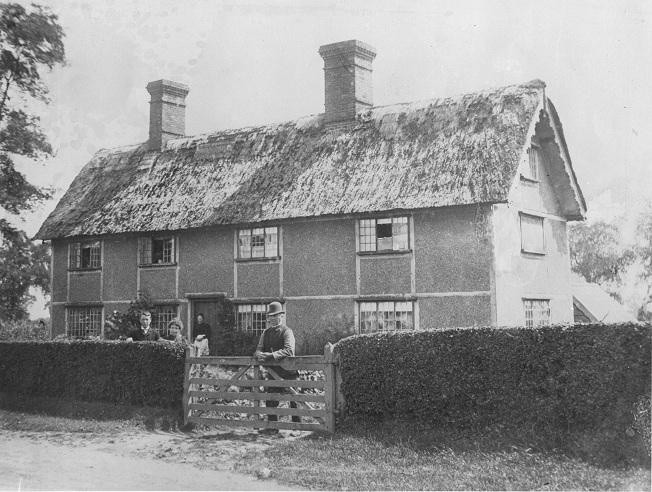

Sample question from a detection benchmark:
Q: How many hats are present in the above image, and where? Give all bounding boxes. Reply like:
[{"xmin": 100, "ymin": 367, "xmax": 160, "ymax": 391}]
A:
[{"xmin": 266, "ymin": 301, "xmax": 285, "ymax": 316}]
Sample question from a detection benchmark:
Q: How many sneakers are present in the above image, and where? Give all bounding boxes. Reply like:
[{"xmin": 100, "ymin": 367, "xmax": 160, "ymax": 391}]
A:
[{"xmin": 259, "ymin": 427, "xmax": 301, "ymax": 436}]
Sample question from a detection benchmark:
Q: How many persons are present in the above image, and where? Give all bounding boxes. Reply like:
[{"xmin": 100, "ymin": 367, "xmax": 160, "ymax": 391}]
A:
[
  {"xmin": 191, "ymin": 312, "xmax": 213, "ymax": 357},
  {"xmin": 255, "ymin": 301, "xmax": 301, "ymax": 436},
  {"xmin": 125, "ymin": 310, "xmax": 186, "ymax": 344}
]
